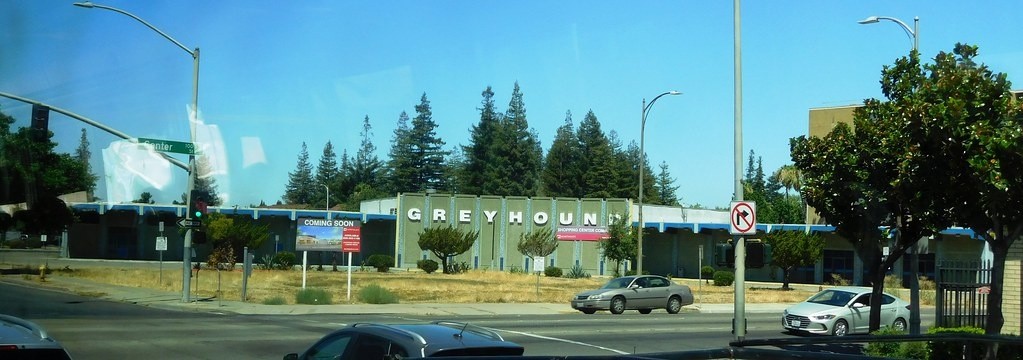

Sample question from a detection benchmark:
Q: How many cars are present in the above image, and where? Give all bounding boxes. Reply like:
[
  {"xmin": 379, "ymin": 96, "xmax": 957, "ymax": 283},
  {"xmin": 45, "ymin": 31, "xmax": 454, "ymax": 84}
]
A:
[
  {"xmin": 571, "ymin": 275, "xmax": 694, "ymax": 315},
  {"xmin": 782, "ymin": 287, "xmax": 922, "ymax": 337}
]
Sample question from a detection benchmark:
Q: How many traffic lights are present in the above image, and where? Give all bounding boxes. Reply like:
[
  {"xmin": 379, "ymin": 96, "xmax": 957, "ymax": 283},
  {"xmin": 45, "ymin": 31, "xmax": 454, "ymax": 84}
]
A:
[{"xmin": 189, "ymin": 189, "xmax": 208, "ymax": 219}]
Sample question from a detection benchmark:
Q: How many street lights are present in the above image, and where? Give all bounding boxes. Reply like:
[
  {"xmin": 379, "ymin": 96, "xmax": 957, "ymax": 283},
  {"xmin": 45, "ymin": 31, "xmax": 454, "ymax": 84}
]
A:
[
  {"xmin": 0, "ymin": 2, "xmax": 200, "ymax": 301},
  {"xmin": 321, "ymin": 183, "xmax": 328, "ymax": 210},
  {"xmin": 636, "ymin": 90, "xmax": 684, "ymax": 276},
  {"xmin": 860, "ymin": 16, "xmax": 919, "ymax": 340}
]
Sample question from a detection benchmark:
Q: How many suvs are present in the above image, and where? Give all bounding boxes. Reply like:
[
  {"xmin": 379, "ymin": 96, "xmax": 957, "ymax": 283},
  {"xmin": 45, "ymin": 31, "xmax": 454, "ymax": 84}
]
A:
[
  {"xmin": 283, "ymin": 320, "xmax": 525, "ymax": 360},
  {"xmin": 0, "ymin": 314, "xmax": 71, "ymax": 360}
]
